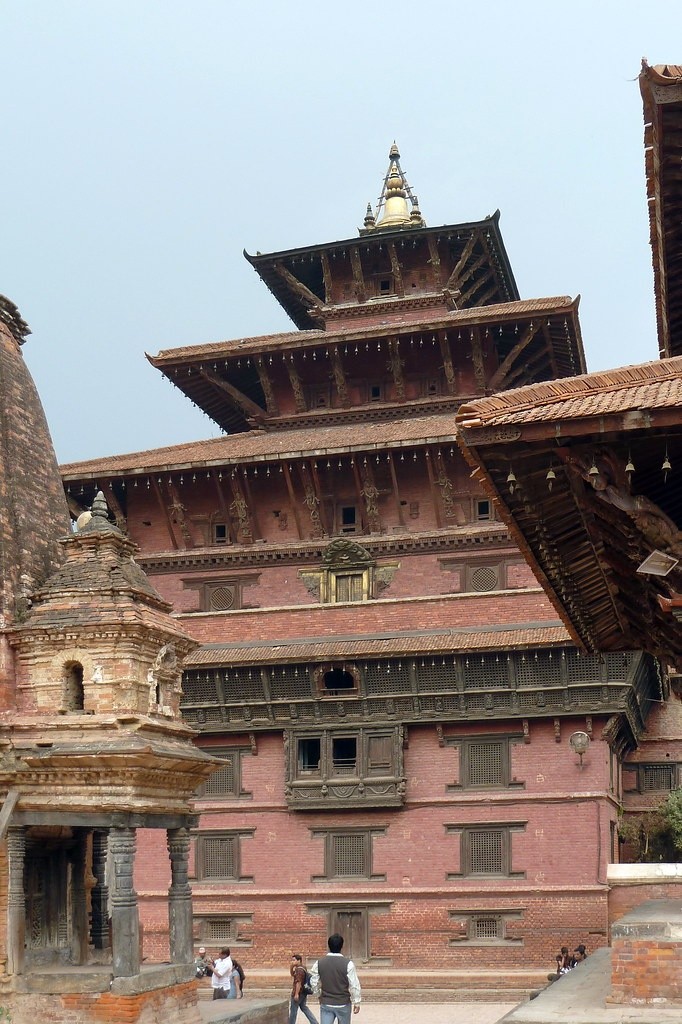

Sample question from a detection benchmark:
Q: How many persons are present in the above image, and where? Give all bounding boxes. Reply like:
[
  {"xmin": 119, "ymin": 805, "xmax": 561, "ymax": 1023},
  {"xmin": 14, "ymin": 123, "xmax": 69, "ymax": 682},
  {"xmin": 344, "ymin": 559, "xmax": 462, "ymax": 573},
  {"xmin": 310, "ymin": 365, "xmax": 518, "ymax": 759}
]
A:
[
  {"xmin": 227, "ymin": 960, "xmax": 246, "ymax": 999},
  {"xmin": 289, "ymin": 955, "xmax": 320, "ymax": 1024},
  {"xmin": 205, "ymin": 946, "xmax": 233, "ymax": 1001},
  {"xmin": 570, "ymin": 944, "xmax": 587, "ymax": 969},
  {"xmin": 309, "ymin": 933, "xmax": 361, "ymax": 1024},
  {"xmin": 194, "ymin": 947, "xmax": 215, "ymax": 979},
  {"xmin": 547, "ymin": 946, "xmax": 571, "ymax": 981}
]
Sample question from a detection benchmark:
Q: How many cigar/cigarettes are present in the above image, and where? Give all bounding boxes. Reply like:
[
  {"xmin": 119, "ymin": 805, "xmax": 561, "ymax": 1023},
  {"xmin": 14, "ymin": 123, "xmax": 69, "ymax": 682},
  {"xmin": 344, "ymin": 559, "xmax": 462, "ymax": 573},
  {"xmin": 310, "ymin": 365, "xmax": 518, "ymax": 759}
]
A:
[{"xmin": 201, "ymin": 974, "xmax": 202, "ymax": 975}]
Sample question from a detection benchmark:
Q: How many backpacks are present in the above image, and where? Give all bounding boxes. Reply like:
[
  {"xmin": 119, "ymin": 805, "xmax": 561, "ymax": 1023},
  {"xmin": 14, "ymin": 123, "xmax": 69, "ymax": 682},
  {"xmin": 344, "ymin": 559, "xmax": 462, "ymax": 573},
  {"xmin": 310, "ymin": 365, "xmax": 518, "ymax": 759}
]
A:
[{"xmin": 297, "ymin": 965, "xmax": 314, "ymax": 995}]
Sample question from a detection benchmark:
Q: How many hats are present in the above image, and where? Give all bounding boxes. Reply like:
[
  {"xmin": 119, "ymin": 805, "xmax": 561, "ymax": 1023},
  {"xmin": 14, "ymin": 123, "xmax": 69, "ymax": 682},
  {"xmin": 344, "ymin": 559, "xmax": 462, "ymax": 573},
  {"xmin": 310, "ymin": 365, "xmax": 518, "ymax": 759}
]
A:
[{"xmin": 199, "ymin": 948, "xmax": 205, "ymax": 953}]
